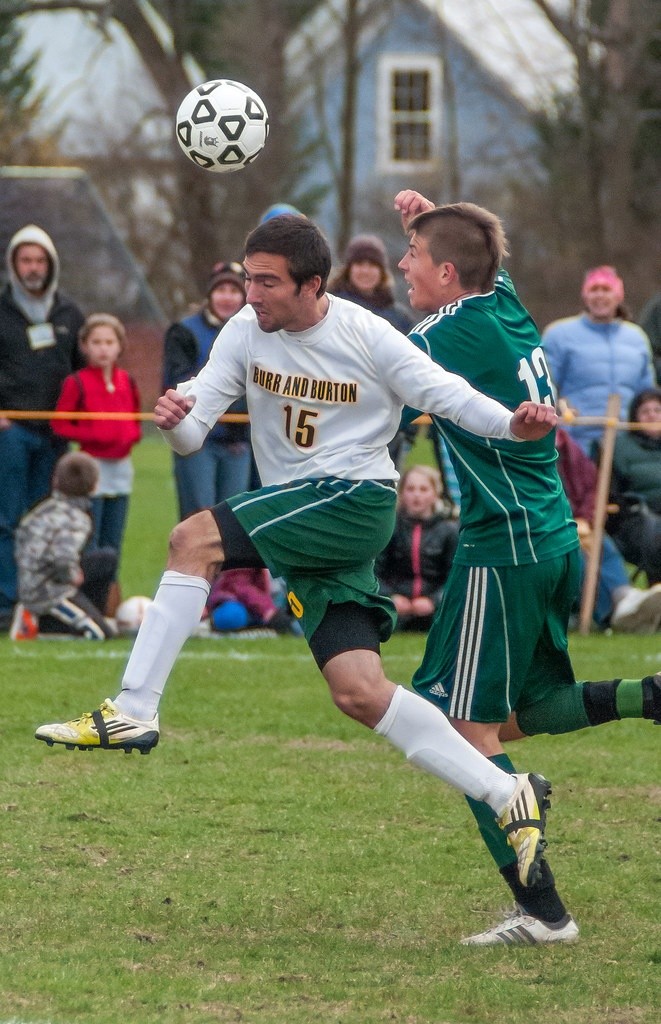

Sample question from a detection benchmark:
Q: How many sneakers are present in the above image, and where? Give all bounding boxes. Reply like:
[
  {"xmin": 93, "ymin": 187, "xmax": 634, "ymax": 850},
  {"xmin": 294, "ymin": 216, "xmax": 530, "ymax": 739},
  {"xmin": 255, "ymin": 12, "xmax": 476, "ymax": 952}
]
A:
[
  {"xmin": 458, "ymin": 898, "xmax": 580, "ymax": 949},
  {"xmin": 35, "ymin": 697, "xmax": 160, "ymax": 755},
  {"xmin": 9, "ymin": 603, "xmax": 39, "ymax": 642},
  {"xmin": 610, "ymin": 582, "xmax": 661, "ymax": 634},
  {"xmin": 494, "ymin": 771, "xmax": 552, "ymax": 887},
  {"xmin": 642, "ymin": 671, "xmax": 661, "ymax": 726}
]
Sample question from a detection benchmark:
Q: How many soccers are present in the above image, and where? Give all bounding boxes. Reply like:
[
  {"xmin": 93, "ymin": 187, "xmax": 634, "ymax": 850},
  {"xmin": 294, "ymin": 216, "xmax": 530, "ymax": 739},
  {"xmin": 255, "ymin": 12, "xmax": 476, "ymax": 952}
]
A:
[{"xmin": 175, "ymin": 80, "xmax": 270, "ymax": 172}]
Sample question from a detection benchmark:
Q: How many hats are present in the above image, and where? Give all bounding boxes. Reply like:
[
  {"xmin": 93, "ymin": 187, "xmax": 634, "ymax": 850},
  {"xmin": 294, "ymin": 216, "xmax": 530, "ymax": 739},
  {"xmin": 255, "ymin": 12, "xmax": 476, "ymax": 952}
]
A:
[
  {"xmin": 206, "ymin": 261, "xmax": 246, "ymax": 303},
  {"xmin": 77, "ymin": 314, "xmax": 126, "ymax": 357},
  {"xmin": 344, "ymin": 236, "xmax": 386, "ymax": 268}
]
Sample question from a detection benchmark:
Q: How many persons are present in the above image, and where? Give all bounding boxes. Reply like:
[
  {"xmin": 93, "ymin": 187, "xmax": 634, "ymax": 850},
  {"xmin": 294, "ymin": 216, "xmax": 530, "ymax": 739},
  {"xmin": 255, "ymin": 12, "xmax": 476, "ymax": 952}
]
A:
[
  {"xmin": 541, "ymin": 264, "xmax": 656, "ymax": 452},
  {"xmin": 50, "ymin": 313, "xmax": 142, "ymax": 612},
  {"xmin": 0, "ymin": 224, "xmax": 89, "ymax": 636},
  {"xmin": 590, "ymin": 388, "xmax": 661, "ymax": 585},
  {"xmin": 35, "ymin": 214, "xmax": 559, "ymax": 885},
  {"xmin": 554, "ymin": 429, "xmax": 661, "ymax": 631},
  {"xmin": 160, "ymin": 261, "xmax": 252, "ymax": 519},
  {"xmin": 10, "ymin": 451, "xmax": 113, "ymax": 640},
  {"xmin": 373, "ymin": 464, "xmax": 460, "ymax": 632},
  {"xmin": 331, "ymin": 234, "xmax": 420, "ymax": 465},
  {"xmin": 202, "ymin": 567, "xmax": 305, "ymax": 637},
  {"xmin": 394, "ymin": 189, "xmax": 661, "ymax": 942}
]
